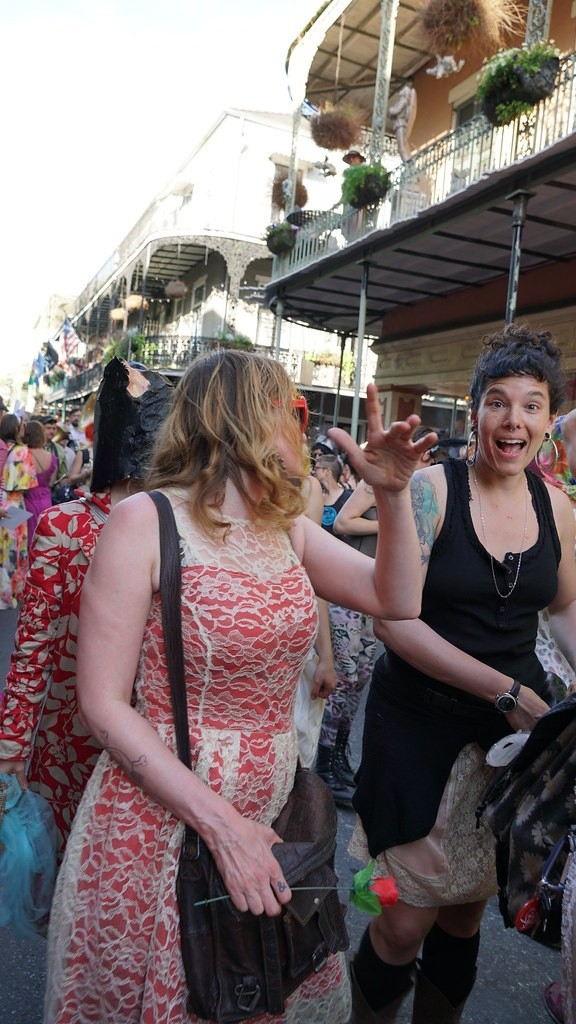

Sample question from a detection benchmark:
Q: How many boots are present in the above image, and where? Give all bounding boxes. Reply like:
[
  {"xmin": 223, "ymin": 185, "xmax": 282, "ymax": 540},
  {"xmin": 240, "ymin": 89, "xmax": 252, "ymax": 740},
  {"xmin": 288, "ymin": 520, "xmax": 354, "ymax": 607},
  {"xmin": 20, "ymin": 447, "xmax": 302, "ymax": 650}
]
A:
[
  {"xmin": 347, "ymin": 961, "xmax": 415, "ymax": 1024},
  {"xmin": 410, "ymin": 956, "xmax": 478, "ymax": 1024}
]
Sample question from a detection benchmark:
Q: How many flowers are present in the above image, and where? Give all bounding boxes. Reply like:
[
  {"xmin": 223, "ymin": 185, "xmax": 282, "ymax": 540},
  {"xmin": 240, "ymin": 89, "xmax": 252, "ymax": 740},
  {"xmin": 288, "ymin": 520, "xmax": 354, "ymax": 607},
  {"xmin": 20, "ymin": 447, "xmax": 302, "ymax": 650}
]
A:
[
  {"xmin": 471, "ymin": 32, "xmax": 566, "ymax": 106},
  {"xmin": 190, "ymin": 851, "xmax": 399, "ymax": 918},
  {"xmin": 258, "ymin": 217, "xmax": 290, "ymax": 241}
]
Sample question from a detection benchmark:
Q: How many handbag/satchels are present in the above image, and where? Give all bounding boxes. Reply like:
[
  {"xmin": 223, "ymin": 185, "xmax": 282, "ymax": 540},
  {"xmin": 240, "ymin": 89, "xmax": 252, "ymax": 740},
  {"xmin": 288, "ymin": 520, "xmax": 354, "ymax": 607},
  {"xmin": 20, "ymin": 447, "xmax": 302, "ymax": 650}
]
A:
[
  {"xmin": 173, "ymin": 767, "xmax": 351, "ymax": 1024},
  {"xmin": 481, "ymin": 696, "xmax": 576, "ymax": 951},
  {"xmin": 56, "ymin": 486, "xmax": 79, "ymax": 502}
]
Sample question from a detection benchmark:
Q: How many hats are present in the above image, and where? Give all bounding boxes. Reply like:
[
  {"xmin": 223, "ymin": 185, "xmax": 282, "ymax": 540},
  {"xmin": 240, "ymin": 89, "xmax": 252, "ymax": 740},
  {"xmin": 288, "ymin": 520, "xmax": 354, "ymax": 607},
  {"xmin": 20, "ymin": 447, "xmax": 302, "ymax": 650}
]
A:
[{"xmin": 39, "ymin": 416, "xmax": 58, "ymax": 426}]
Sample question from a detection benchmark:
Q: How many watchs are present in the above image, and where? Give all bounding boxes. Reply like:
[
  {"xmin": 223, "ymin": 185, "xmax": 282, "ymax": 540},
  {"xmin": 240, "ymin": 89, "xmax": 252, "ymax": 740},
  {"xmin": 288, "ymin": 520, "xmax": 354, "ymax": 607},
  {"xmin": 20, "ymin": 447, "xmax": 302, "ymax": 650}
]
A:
[{"xmin": 495, "ymin": 679, "xmax": 521, "ymax": 714}]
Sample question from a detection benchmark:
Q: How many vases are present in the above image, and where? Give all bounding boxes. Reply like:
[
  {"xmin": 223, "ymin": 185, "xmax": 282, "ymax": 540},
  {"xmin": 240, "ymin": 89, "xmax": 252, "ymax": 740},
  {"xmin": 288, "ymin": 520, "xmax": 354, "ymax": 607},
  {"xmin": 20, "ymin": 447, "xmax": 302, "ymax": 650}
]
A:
[
  {"xmin": 267, "ymin": 229, "xmax": 295, "ymax": 256},
  {"xmin": 481, "ymin": 54, "xmax": 560, "ymax": 128}
]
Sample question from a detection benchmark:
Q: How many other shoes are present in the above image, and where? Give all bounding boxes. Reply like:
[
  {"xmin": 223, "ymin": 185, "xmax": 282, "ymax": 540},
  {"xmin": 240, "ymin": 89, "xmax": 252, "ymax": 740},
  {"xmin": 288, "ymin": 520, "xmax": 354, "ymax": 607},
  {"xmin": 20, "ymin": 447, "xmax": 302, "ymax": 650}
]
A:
[
  {"xmin": 543, "ymin": 981, "xmax": 568, "ymax": 1024},
  {"xmin": 0, "ymin": 598, "xmax": 17, "ymax": 610}
]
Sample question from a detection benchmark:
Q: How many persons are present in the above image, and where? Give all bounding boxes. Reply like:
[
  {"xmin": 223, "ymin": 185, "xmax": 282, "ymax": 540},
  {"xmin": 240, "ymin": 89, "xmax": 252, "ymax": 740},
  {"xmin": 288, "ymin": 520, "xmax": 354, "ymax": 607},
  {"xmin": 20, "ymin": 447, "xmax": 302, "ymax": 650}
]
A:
[
  {"xmin": 314, "ymin": 155, "xmax": 337, "ymax": 178},
  {"xmin": 0, "ymin": 358, "xmax": 179, "ymax": 939},
  {"xmin": 426, "ymin": 54, "xmax": 465, "ymax": 78},
  {"xmin": 349, "ymin": 318, "xmax": 576, "ymax": 1024},
  {"xmin": 526, "ymin": 407, "xmax": 575, "ymax": 502},
  {"xmin": 46, "ymin": 350, "xmax": 439, "ymax": 1024},
  {"xmin": 389, "ymin": 84, "xmax": 416, "ymax": 168},
  {"xmin": 333, "ymin": 150, "xmax": 366, "ymax": 241},
  {"xmin": 0, "ymin": 396, "xmax": 96, "ymax": 611},
  {"xmin": 292, "ymin": 427, "xmax": 385, "ymax": 797}
]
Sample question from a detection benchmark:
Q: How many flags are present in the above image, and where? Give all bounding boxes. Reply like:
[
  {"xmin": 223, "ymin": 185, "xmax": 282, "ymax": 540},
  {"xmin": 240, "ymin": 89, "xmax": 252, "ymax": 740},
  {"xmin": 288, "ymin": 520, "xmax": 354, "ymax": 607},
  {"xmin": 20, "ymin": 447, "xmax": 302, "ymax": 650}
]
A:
[{"xmin": 63, "ymin": 314, "xmax": 82, "ymax": 357}]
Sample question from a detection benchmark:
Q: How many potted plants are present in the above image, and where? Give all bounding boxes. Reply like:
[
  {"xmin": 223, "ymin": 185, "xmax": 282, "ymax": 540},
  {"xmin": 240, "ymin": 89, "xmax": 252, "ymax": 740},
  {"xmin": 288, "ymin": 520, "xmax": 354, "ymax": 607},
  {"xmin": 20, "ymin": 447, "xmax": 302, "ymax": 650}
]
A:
[
  {"xmin": 101, "ymin": 329, "xmax": 159, "ymax": 368},
  {"xmin": 341, "ymin": 160, "xmax": 393, "ymax": 210}
]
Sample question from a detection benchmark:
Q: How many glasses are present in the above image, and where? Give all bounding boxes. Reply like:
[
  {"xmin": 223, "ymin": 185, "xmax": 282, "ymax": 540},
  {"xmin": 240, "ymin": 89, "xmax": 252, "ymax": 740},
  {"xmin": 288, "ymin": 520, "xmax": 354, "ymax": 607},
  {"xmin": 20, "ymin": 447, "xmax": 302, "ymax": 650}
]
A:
[
  {"xmin": 270, "ymin": 392, "xmax": 308, "ymax": 433},
  {"xmin": 314, "ymin": 452, "xmax": 321, "ymax": 458},
  {"xmin": 314, "ymin": 465, "xmax": 329, "ymax": 473}
]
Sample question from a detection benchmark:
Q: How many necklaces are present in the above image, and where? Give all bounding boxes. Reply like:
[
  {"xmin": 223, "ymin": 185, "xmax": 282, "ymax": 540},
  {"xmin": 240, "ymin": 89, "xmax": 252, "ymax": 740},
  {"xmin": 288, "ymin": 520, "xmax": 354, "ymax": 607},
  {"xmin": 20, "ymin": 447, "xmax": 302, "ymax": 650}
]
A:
[{"xmin": 470, "ymin": 467, "xmax": 529, "ymax": 598}]
